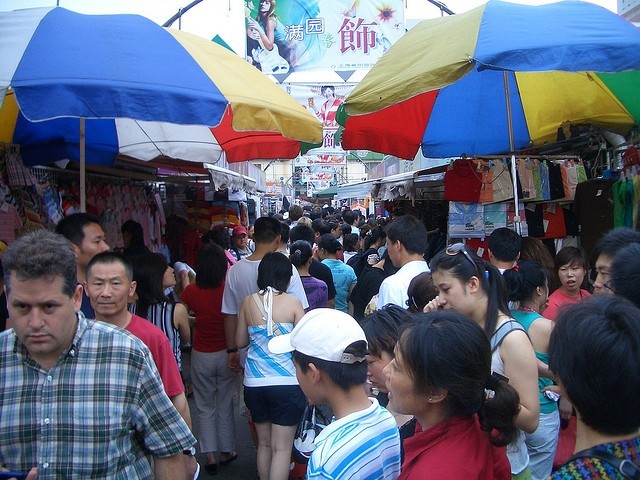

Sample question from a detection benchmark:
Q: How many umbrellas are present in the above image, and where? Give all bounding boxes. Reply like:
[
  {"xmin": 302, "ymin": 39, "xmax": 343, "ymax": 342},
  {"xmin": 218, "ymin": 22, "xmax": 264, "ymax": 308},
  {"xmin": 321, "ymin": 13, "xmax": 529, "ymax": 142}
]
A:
[
  {"xmin": 0, "ymin": 6, "xmax": 324, "ymax": 215},
  {"xmin": 333, "ymin": 0, "xmax": 640, "ymax": 236}
]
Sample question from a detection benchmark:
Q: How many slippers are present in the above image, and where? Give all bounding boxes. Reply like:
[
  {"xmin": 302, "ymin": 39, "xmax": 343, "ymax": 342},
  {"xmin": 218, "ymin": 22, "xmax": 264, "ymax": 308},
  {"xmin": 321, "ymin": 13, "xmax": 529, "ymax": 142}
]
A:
[
  {"xmin": 220, "ymin": 452, "xmax": 237, "ymax": 465},
  {"xmin": 204, "ymin": 459, "xmax": 217, "ymax": 471}
]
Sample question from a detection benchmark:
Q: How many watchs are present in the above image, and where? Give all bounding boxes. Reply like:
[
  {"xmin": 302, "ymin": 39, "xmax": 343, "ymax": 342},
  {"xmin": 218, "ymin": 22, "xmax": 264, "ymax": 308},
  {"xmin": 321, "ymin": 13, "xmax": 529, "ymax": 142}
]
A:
[{"xmin": 183, "ymin": 446, "xmax": 196, "ymax": 457}]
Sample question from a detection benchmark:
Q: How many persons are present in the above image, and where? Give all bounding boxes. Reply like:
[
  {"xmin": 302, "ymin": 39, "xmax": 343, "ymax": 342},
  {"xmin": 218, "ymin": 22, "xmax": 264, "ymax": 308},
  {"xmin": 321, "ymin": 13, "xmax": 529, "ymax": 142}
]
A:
[
  {"xmin": 288, "ymin": 241, "xmax": 329, "ymax": 311},
  {"xmin": 516, "ymin": 238, "xmax": 563, "ymax": 287},
  {"xmin": 359, "ymin": 302, "xmax": 416, "ymax": 465},
  {"xmin": 342, "ymin": 235, "xmax": 360, "ymax": 260},
  {"xmin": 408, "ymin": 272, "xmax": 440, "ymax": 312},
  {"xmin": 129, "ymin": 253, "xmax": 192, "ymax": 389},
  {"xmin": 166, "ymin": 211, "xmax": 225, "ymax": 258},
  {"xmin": 276, "ymin": 225, "xmax": 291, "ymax": 254},
  {"xmin": 364, "ymin": 215, "xmax": 431, "ymax": 316},
  {"xmin": 247, "ymin": 0, "xmax": 308, "ymax": 69},
  {"xmin": 590, "ymin": 227, "xmax": 640, "ymax": 295},
  {"xmin": 290, "ymin": 226, "xmax": 336, "ymax": 311},
  {"xmin": 608, "ymin": 242, "xmax": 640, "ymax": 307},
  {"xmin": 310, "ymin": 86, "xmax": 345, "ymax": 128},
  {"xmin": 88, "ymin": 251, "xmax": 201, "ymax": 480},
  {"xmin": 505, "ymin": 260, "xmax": 561, "ymax": 480},
  {"xmin": 1, "ymin": 230, "xmax": 198, "ymax": 480},
  {"xmin": 362, "ymin": 231, "xmax": 389, "ymax": 266},
  {"xmin": 120, "ymin": 219, "xmax": 168, "ymax": 312},
  {"xmin": 256, "ymin": 199, "xmax": 392, "ymax": 235},
  {"xmin": 543, "ymin": 246, "xmax": 591, "ymax": 321},
  {"xmin": 382, "ymin": 309, "xmax": 512, "ymax": 480},
  {"xmin": 487, "ymin": 227, "xmax": 522, "ymax": 275},
  {"xmin": 423, "ymin": 242, "xmax": 541, "ymax": 480},
  {"xmin": 267, "ymin": 307, "xmax": 401, "ymax": 480},
  {"xmin": 236, "ymin": 252, "xmax": 305, "ymax": 479},
  {"xmin": 249, "ymin": 223, "xmax": 257, "ymax": 240},
  {"xmin": 181, "ymin": 243, "xmax": 238, "ymax": 474},
  {"xmin": 219, "ymin": 216, "xmax": 308, "ymax": 374},
  {"xmin": 316, "ymin": 233, "xmax": 359, "ymax": 314},
  {"xmin": 543, "ymin": 291, "xmax": 640, "ymax": 480},
  {"xmin": 225, "ymin": 226, "xmax": 257, "ymax": 260},
  {"xmin": 56, "ymin": 214, "xmax": 112, "ymax": 319}
]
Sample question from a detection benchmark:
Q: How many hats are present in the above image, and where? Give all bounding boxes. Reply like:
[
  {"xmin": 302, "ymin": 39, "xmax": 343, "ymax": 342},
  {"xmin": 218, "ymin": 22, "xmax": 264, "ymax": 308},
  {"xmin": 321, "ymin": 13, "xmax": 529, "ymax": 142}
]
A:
[
  {"xmin": 232, "ymin": 226, "xmax": 246, "ymax": 237},
  {"xmin": 267, "ymin": 308, "xmax": 369, "ymax": 365}
]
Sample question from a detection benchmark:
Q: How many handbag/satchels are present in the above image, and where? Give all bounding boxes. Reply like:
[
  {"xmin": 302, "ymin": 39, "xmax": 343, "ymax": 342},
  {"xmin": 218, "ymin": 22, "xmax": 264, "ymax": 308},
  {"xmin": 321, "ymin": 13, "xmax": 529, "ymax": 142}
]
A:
[
  {"xmin": 293, "ymin": 413, "xmax": 330, "ymax": 464},
  {"xmin": 555, "ymin": 415, "xmax": 578, "ymax": 467}
]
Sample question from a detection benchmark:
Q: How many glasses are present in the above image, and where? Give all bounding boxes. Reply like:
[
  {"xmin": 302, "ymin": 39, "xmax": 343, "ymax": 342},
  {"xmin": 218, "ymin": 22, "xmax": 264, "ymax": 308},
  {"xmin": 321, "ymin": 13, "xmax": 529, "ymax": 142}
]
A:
[
  {"xmin": 603, "ymin": 279, "xmax": 616, "ymax": 295},
  {"xmin": 445, "ymin": 242, "xmax": 478, "ymax": 271}
]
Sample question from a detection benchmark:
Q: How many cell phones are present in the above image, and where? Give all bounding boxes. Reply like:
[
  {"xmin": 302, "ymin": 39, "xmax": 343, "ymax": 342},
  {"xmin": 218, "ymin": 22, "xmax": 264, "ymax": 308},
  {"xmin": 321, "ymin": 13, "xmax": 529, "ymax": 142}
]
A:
[{"xmin": 4, "ymin": 470, "xmax": 27, "ymax": 479}]
